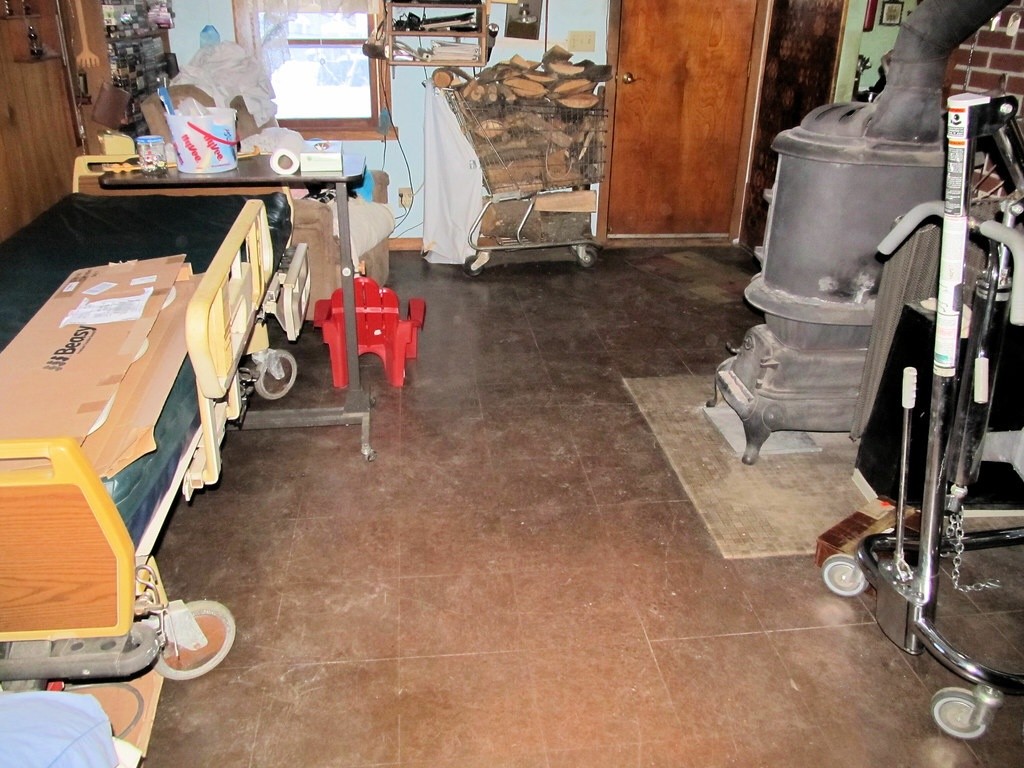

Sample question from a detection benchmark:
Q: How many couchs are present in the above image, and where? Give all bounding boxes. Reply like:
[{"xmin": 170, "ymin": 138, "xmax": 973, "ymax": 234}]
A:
[{"xmin": 139, "ymin": 73, "xmax": 394, "ymax": 326}]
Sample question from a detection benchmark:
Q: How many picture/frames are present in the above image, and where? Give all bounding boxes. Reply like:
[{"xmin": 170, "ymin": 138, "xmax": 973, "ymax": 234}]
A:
[
  {"xmin": 504, "ymin": 0, "xmax": 543, "ymax": 40},
  {"xmin": 879, "ymin": 0, "xmax": 904, "ymax": 26}
]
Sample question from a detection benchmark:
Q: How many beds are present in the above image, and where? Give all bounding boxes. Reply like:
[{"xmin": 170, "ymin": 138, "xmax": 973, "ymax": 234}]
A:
[{"xmin": 1, "ymin": 153, "xmax": 313, "ymax": 680}]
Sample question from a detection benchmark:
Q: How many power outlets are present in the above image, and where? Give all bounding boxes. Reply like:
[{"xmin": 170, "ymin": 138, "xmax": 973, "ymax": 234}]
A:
[{"xmin": 398, "ymin": 188, "xmax": 413, "ymax": 208}]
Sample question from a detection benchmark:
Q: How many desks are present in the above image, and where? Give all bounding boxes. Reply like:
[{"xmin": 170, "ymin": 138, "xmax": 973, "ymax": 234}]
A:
[{"xmin": 95, "ymin": 140, "xmax": 379, "ymax": 464}]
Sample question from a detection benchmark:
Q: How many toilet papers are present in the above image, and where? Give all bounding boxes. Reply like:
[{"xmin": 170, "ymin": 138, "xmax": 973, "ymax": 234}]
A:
[{"xmin": 270, "ymin": 131, "xmax": 304, "ymax": 175}]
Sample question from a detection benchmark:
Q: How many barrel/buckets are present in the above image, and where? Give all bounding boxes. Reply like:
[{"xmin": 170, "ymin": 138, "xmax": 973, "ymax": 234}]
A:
[{"xmin": 164, "ymin": 107, "xmax": 240, "ymax": 174}]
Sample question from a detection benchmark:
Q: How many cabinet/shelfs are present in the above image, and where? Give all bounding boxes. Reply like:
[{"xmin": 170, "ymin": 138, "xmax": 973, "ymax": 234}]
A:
[
  {"xmin": 0, "ymin": 0, "xmax": 60, "ymax": 63},
  {"xmin": 386, "ymin": 3, "xmax": 488, "ymax": 67}
]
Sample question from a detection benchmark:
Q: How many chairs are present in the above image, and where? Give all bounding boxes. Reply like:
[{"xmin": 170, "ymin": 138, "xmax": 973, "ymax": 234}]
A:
[{"xmin": 314, "ymin": 276, "xmax": 427, "ymax": 390}]
